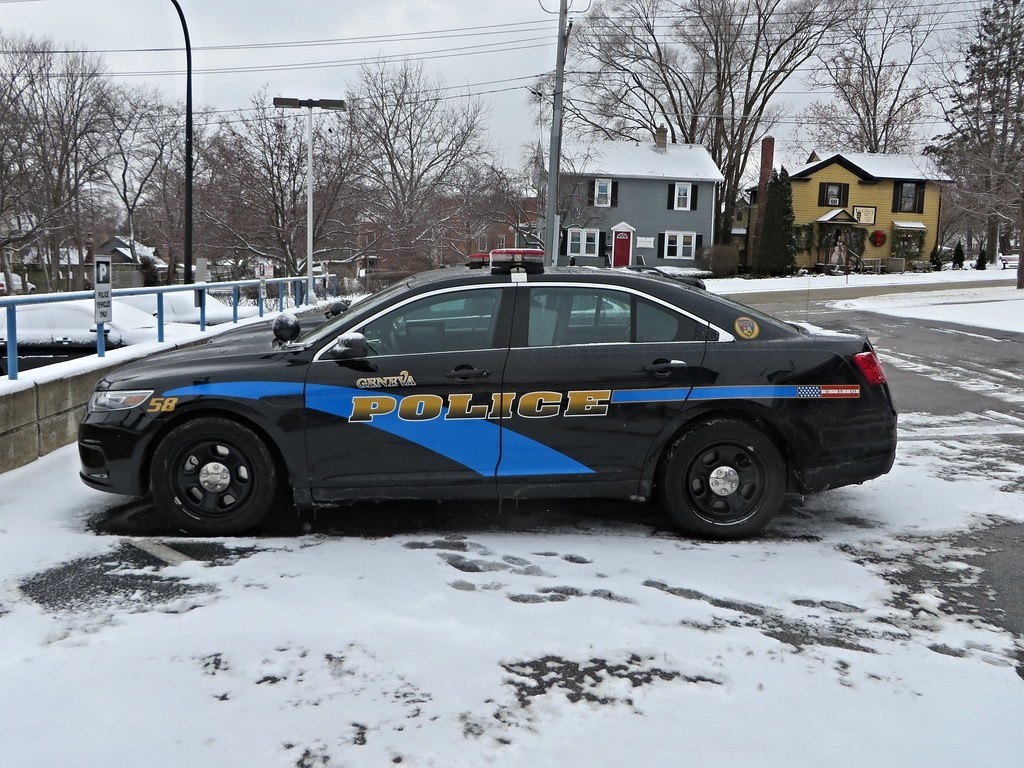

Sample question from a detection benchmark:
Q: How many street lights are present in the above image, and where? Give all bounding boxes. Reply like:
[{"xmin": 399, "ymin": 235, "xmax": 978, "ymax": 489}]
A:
[{"xmin": 272, "ymin": 97, "xmax": 347, "ymax": 291}]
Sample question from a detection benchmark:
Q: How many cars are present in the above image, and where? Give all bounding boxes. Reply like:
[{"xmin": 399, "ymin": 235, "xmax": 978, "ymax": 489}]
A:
[{"xmin": 0, "ymin": 247, "xmax": 898, "ymax": 542}]
